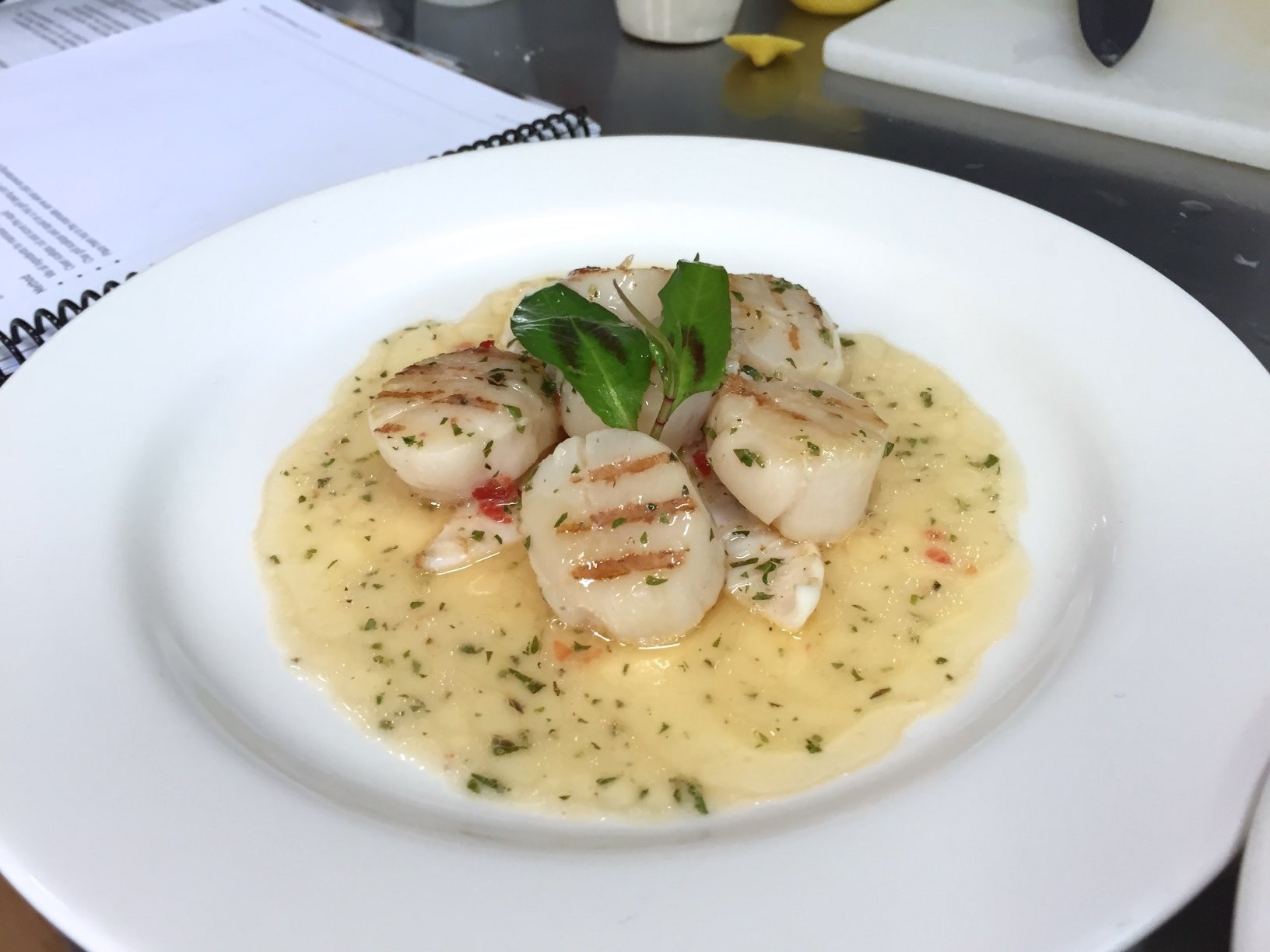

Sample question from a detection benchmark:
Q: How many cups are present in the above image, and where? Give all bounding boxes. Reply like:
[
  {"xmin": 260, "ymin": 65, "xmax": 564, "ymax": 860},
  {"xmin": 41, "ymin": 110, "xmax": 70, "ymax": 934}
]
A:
[{"xmin": 612, "ymin": 1, "xmax": 743, "ymax": 48}]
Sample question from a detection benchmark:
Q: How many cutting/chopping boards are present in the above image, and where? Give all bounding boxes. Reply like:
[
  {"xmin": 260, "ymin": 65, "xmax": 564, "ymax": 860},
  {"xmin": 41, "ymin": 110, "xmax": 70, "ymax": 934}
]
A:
[{"xmin": 822, "ymin": 1, "xmax": 1270, "ymax": 169}]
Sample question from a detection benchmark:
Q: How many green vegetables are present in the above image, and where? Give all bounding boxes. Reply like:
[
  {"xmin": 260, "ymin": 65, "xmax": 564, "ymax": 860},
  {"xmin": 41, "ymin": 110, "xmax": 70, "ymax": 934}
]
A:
[{"xmin": 510, "ymin": 252, "xmax": 734, "ymax": 441}]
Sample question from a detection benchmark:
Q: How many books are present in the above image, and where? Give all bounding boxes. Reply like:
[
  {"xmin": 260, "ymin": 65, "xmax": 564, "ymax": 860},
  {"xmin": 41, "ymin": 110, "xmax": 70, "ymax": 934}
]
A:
[{"xmin": 0, "ymin": 3, "xmax": 601, "ymax": 379}]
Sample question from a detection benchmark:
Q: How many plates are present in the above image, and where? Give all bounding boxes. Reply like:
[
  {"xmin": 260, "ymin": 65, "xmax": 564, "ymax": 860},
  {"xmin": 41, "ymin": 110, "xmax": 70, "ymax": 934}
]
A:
[{"xmin": 3, "ymin": 132, "xmax": 1270, "ymax": 952}]
